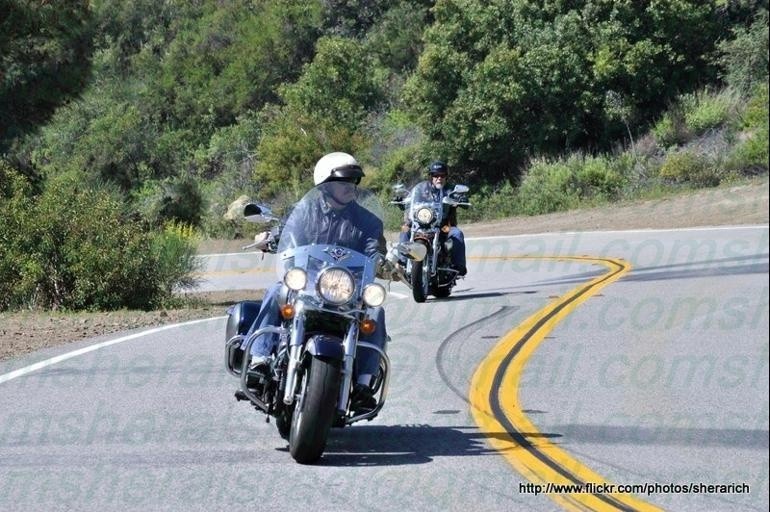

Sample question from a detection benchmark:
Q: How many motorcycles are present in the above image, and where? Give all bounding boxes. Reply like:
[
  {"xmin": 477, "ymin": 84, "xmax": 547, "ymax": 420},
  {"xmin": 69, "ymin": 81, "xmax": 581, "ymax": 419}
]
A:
[
  {"xmin": 226, "ymin": 180, "xmax": 404, "ymax": 465},
  {"xmin": 389, "ymin": 177, "xmax": 473, "ymax": 302}
]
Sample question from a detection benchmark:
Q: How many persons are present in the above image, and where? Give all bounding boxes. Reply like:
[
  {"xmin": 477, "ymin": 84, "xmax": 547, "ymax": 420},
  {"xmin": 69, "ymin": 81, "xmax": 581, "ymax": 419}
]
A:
[
  {"xmin": 240, "ymin": 150, "xmax": 386, "ymax": 414},
  {"xmin": 397, "ymin": 160, "xmax": 467, "ymax": 277}
]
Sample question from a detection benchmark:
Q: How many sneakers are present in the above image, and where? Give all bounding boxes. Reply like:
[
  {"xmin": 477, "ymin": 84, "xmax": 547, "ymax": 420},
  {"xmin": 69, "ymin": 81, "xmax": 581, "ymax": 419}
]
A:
[
  {"xmin": 451, "ymin": 266, "xmax": 466, "ymax": 276},
  {"xmin": 393, "ymin": 254, "xmax": 406, "ymax": 270}
]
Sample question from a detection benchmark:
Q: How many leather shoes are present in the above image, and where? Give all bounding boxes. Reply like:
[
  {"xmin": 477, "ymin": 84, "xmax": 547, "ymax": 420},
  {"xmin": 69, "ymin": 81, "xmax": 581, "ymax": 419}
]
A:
[
  {"xmin": 246, "ymin": 362, "xmax": 271, "ymax": 389},
  {"xmin": 349, "ymin": 388, "xmax": 377, "ymax": 411}
]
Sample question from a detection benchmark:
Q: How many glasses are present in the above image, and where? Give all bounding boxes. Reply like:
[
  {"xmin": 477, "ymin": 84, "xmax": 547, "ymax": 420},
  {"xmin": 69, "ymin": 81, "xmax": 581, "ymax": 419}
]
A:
[
  {"xmin": 330, "ymin": 177, "xmax": 358, "ymax": 184},
  {"xmin": 432, "ymin": 174, "xmax": 448, "ymax": 178}
]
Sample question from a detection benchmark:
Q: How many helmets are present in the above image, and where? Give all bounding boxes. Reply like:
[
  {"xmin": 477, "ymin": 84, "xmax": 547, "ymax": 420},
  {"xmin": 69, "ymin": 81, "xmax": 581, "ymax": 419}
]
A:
[
  {"xmin": 428, "ymin": 161, "xmax": 449, "ymax": 176},
  {"xmin": 314, "ymin": 151, "xmax": 365, "ymax": 187}
]
